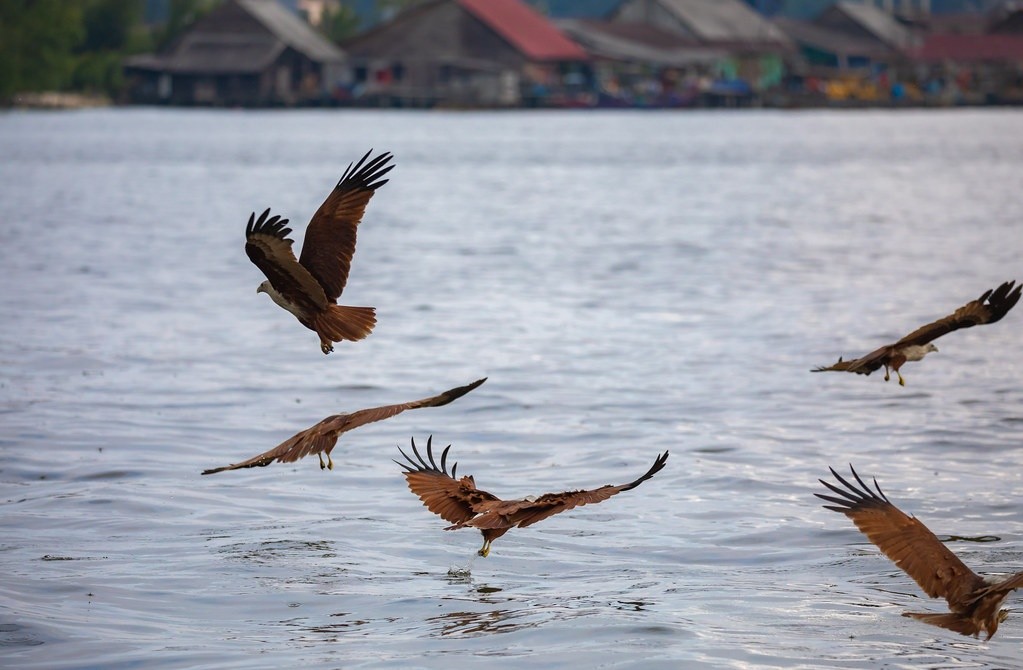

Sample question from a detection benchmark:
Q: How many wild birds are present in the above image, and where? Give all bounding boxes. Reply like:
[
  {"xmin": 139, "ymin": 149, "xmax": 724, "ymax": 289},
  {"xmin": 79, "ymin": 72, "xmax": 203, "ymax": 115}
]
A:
[
  {"xmin": 245, "ymin": 148, "xmax": 396, "ymax": 354},
  {"xmin": 810, "ymin": 280, "xmax": 1023, "ymax": 387},
  {"xmin": 200, "ymin": 377, "xmax": 489, "ymax": 476},
  {"xmin": 814, "ymin": 463, "xmax": 1023, "ymax": 642},
  {"xmin": 392, "ymin": 434, "xmax": 669, "ymax": 558}
]
